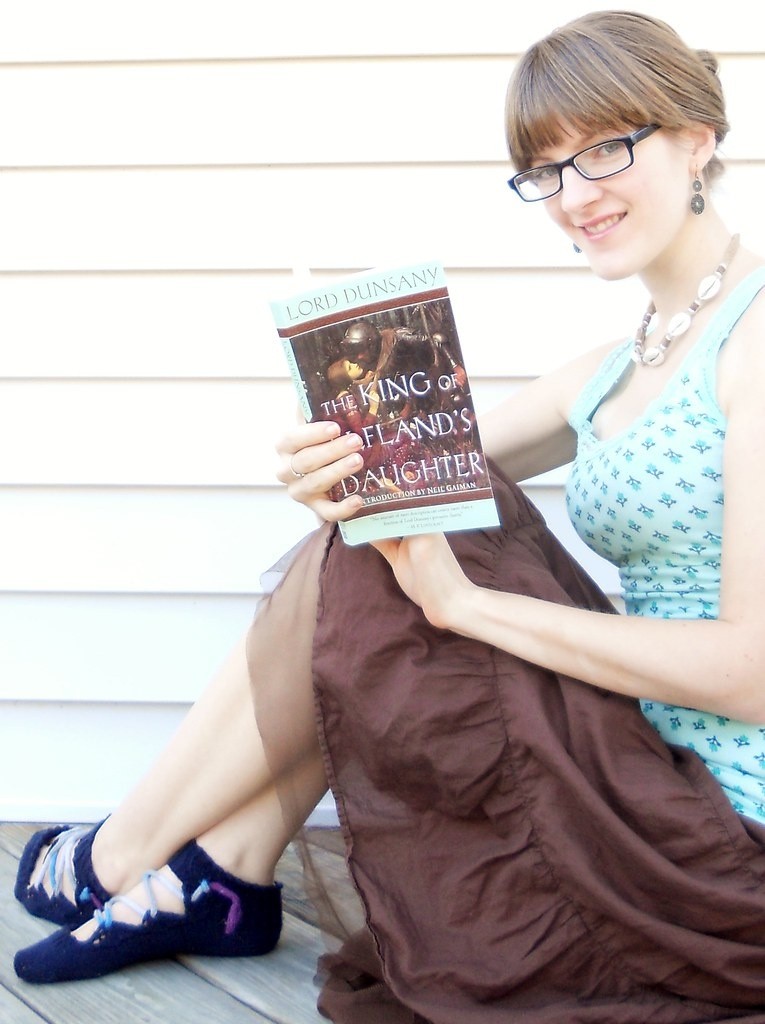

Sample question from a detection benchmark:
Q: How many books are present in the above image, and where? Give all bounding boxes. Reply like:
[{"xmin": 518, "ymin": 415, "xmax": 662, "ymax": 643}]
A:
[{"xmin": 269, "ymin": 244, "xmax": 501, "ymax": 547}]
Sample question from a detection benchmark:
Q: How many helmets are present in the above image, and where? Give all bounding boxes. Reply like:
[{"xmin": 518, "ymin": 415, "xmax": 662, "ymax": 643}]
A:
[{"xmin": 340, "ymin": 322, "xmax": 379, "ymax": 350}]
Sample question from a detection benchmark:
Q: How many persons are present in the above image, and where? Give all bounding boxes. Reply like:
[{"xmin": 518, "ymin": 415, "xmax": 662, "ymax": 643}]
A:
[{"xmin": 11, "ymin": 12, "xmax": 765, "ymax": 1024}]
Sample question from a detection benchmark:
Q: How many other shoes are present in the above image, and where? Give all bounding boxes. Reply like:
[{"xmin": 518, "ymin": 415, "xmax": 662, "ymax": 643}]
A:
[
  {"xmin": 15, "ymin": 837, "xmax": 290, "ymax": 984},
  {"xmin": 14, "ymin": 813, "xmax": 116, "ymax": 924}
]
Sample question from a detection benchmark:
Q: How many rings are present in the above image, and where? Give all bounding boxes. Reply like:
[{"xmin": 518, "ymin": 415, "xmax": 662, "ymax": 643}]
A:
[{"xmin": 289, "ymin": 453, "xmax": 307, "ymax": 476}]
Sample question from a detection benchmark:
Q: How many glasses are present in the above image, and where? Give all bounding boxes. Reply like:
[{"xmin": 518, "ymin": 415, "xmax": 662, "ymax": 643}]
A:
[{"xmin": 508, "ymin": 121, "xmax": 659, "ymax": 201}]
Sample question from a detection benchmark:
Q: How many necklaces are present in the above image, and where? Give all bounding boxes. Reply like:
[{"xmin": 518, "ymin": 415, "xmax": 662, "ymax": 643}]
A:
[{"xmin": 631, "ymin": 231, "xmax": 740, "ymax": 367}]
